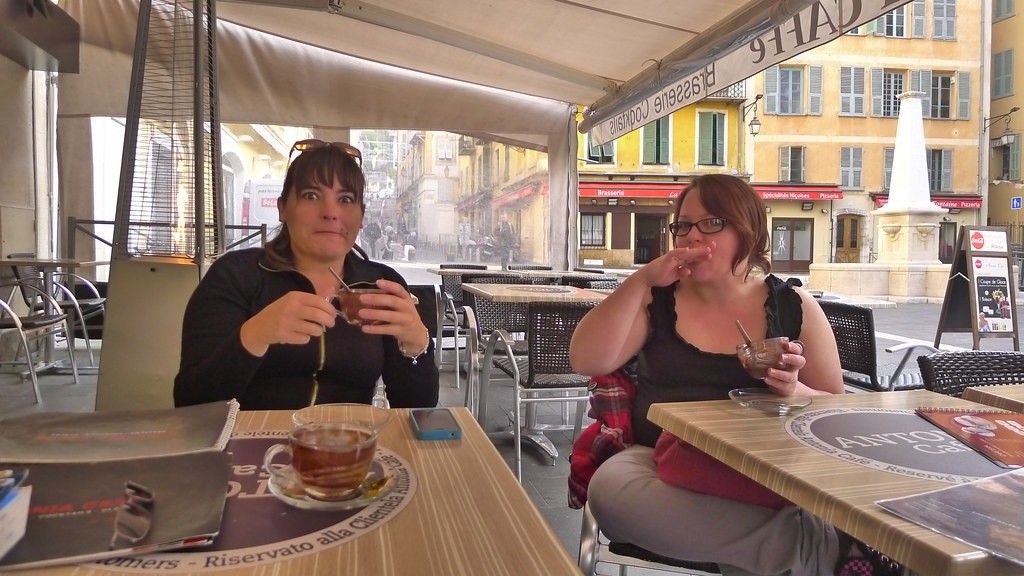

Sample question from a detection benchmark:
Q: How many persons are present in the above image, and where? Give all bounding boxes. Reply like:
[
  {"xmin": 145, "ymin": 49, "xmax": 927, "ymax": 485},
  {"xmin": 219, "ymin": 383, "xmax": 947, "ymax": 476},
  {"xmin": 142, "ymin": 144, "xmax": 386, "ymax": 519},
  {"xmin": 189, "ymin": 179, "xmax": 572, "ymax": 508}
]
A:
[
  {"xmin": 569, "ymin": 173, "xmax": 900, "ymax": 576},
  {"xmin": 173, "ymin": 139, "xmax": 439, "ymax": 411},
  {"xmin": 355, "ymin": 215, "xmax": 417, "ymax": 261},
  {"xmin": 494, "ymin": 213, "xmax": 519, "ymax": 271}
]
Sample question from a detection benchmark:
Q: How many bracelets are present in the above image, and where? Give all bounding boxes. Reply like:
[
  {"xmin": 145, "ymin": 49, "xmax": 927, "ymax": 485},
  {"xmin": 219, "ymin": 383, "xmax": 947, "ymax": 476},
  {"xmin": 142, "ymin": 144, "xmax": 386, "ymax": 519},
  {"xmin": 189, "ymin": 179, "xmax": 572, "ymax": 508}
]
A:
[{"xmin": 398, "ymin": 323, "xmax": 429, "ymax": 364}]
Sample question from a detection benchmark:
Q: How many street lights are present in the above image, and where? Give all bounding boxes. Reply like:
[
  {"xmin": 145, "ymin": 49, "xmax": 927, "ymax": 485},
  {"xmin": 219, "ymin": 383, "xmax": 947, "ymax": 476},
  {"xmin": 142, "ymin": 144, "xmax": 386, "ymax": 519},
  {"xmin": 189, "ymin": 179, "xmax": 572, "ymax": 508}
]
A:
[{"xmin": 978, "ymin": 107, "xmax": 1020, "ymax": 227}]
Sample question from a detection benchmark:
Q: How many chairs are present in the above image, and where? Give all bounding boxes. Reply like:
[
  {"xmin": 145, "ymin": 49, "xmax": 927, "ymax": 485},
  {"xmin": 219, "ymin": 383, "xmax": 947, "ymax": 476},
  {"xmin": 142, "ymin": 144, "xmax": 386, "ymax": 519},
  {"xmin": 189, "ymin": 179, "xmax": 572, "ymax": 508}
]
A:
[{"xmin": 1, "ymin": 259, "xmax": 1024, "ymax": 576}]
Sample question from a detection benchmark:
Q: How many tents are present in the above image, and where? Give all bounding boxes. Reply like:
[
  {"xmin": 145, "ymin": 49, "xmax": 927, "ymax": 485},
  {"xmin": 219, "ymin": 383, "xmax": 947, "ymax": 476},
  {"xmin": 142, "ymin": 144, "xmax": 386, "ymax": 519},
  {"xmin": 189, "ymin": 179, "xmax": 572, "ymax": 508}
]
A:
[{"xmin": 56, "ymin": 0, "xmax": 578, "ymax": 274}]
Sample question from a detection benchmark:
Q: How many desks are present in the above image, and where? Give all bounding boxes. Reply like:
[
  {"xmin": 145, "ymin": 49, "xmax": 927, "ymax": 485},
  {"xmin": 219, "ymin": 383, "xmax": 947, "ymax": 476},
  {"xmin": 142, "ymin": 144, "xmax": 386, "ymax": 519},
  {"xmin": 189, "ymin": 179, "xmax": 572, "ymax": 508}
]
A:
[
  {"xmin": 426, "ymin": 268, "xmax": 597, "ymax": 385},
  {"xmin": 459, "ymin": 279, "xmax": 611, "ymax": 462},
  {"xmin": 646, "ymin": 389, "xmax": 1023, "ymax": 573},
  {"xmin": 959, "ymin": 384, "xmax": 1023, "ymax": 413},
  {"xmin": 1, "ymin": 258, "xmax": 111, "ymax": 380},
  {"xmin": 1, "ymin": 402, "xmax": 581, "ymax": 575}
]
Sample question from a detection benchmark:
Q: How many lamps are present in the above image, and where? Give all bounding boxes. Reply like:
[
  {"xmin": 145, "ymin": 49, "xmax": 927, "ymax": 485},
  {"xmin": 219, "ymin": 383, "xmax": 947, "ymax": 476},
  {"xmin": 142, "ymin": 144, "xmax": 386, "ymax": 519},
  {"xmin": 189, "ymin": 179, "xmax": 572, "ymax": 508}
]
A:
[
  {"xmin": 741, "ymin": 94, "xmax": 764, "ymax": 136},
  {"xmin": 630, "ymin": 199, "xmax": 635, "ymax": 205},
  {"xmin": 821, "ymin": 208, "xmax": 829, "ymax": 214},
  {"xmin": 766, "ymin": 206, "xmax": 770, "ymax": 214},
  {"xmin": 983, "ymin": 105, "xmax": 1019, "ymax": 145},
  {"xmin": 802, "ymin": 201, "xmax": 815, "ymax": 210},
  {"xmin": 669, "ymin": 200, "xmax": 674, "ymax": 204},
  {"xmin": 592, "ymin": 199, "xmax": 596, "ymax": 204}
]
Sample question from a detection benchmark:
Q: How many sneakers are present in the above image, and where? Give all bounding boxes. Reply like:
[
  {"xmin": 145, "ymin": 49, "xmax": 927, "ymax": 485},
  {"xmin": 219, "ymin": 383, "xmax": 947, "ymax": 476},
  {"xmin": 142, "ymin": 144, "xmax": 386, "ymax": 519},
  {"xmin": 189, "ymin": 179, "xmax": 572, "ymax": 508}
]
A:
[{"xmin": 835, "ymin": 537, "xmax": 909, "ymax": 574}]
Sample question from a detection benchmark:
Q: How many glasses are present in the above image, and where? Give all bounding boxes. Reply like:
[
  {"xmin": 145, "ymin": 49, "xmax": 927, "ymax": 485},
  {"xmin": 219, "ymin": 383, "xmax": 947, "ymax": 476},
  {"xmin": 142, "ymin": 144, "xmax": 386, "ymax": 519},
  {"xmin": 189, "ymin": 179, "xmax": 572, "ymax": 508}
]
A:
[
  {"xmin": 667, "ymin": 212, "xmax": 730, "ymax": 237},
  {"xmin": 284, "ymin": 139, "xmax": 362, "ymax": 180}
]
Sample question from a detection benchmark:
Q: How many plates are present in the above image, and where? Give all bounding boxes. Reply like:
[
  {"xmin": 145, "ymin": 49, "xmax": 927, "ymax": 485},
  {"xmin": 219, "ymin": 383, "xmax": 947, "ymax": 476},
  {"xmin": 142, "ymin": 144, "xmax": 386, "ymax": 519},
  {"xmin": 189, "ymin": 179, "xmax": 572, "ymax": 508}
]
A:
[
  {"xmin": 728, "ymin": 387, "xmax": 813, "ymax": 414},
  {"xmin": 267, "ymin": 457, "xmax": 399, "ymax": 510}
]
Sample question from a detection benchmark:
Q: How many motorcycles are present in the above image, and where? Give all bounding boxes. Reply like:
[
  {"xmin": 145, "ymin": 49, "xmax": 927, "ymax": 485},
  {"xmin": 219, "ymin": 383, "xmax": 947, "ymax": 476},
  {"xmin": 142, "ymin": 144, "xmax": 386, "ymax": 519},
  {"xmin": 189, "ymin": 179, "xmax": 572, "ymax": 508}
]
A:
[{"xmin": 474, "ymin": 236, "xmax": 520, "ymax": 263}]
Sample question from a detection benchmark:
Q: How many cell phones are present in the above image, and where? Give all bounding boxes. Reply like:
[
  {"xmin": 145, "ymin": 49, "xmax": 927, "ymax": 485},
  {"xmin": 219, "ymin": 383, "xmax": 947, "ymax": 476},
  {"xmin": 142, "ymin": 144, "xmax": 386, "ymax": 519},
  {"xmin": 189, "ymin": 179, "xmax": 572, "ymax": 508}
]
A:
[{"xmin": 409, "ymin": 408, "xmax": 462, "ymax": 440}]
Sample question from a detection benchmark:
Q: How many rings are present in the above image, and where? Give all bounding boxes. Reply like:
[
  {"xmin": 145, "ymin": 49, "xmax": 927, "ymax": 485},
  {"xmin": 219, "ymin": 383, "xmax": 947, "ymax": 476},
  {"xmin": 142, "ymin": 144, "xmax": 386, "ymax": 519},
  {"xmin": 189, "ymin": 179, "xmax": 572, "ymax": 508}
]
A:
[
  {"xmin": 674, "ymin": 254, "xmax": 680, "ymax": 261},
  {"xmin": 794, "ymin": 342, "xmax": 803, "ymax": 352}
]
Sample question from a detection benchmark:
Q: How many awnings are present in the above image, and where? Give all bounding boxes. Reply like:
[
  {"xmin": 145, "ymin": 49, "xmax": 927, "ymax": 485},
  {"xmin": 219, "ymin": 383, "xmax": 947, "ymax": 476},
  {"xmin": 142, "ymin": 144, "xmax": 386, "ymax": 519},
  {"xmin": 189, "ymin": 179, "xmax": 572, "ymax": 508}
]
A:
[
  {"xmin": 872, "ymin": 194, "xmax": 983, "ymax": 208},
  {"xmin": 494, "ymin": 185, "xmax": 537, "ymax": 211},
  {"xmin": 158, "ymin": 0, "xmax": 915, "ymax": 147},
  {"xmin": 577, "ymin": 183, "xmax": 844, "ymax": 199}
]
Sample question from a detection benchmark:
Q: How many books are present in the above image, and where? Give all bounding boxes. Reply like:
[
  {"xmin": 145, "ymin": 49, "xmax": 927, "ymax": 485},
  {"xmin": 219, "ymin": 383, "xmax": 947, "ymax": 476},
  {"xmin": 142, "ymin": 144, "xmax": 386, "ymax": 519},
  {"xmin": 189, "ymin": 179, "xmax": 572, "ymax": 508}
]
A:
[
  {"xmin": 914, "ymin": 406, "xmax": 1024, "ymax": 468},
  {"xmin": 0, "ymin": 452, "xmax": 231, "ymax": 568},
  {"xmin": 0, "ymin": 398, "xmax": 240, "ymax": 462}
]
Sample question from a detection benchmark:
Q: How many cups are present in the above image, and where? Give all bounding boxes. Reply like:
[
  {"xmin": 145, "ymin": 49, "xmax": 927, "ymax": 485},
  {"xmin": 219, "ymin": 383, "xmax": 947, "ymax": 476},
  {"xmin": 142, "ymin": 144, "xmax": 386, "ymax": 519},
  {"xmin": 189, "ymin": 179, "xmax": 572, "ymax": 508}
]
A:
[
  {"xmin": 325, "ymin": 287, "xmax": 393, "ymax": 329},
  {"xmin": 262, "ymin": 401, "xmax": 398, "ymax": 499},
  {"xmin": 735, "ymin": 336, "xmax": 805, "ymax": 380}
]
